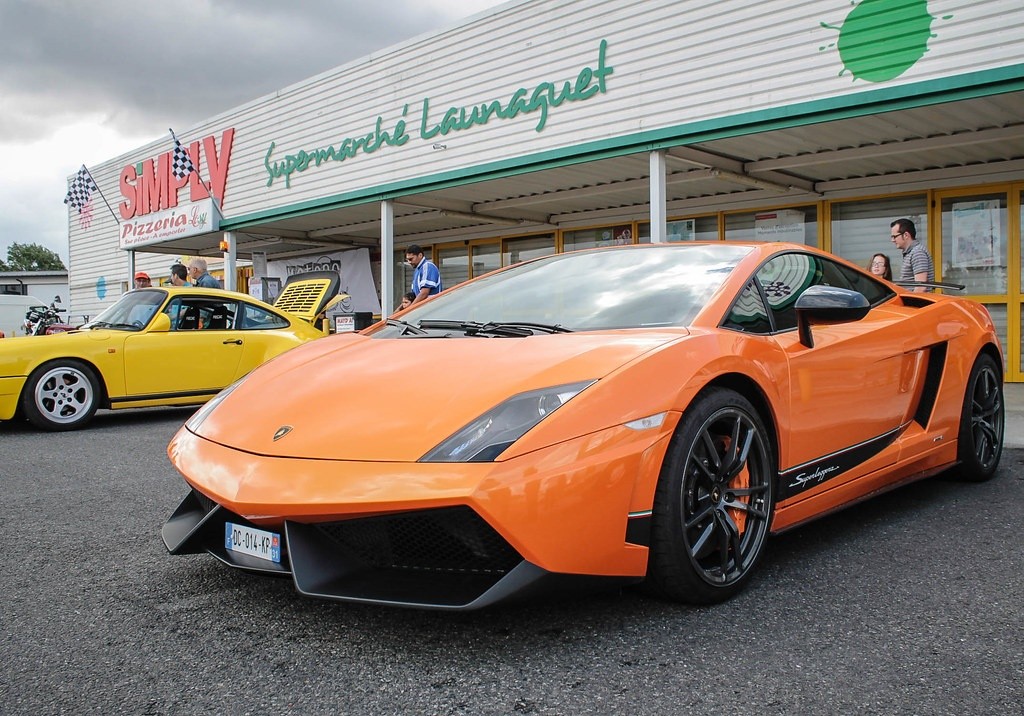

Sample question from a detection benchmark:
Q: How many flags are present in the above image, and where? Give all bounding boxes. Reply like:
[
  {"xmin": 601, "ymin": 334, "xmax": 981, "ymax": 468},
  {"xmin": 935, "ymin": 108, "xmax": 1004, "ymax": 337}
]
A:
[
  {"xmin": 172, "ymin": 131, "xmax": 197, "ymax": 181},
  {"xmin": 64, "ymin": 165, "xmax": 98, "ymax": 211}
]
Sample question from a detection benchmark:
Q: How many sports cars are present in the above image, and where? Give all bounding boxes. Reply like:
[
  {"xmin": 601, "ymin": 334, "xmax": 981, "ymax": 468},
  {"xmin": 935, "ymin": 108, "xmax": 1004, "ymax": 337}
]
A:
[
  {"xmin": 0, "ymin": 269, "xmax": 352, "ymax": 433},
  {"xmin": 160, "ymin": 241, "xmax": 1005, "ymax": 617}
]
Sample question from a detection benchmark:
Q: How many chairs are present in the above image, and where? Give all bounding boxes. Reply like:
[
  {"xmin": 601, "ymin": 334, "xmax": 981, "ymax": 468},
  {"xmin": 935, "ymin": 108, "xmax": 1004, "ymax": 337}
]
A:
[
  {"xmin": 179, "ymin": 305, "xmax": 200, "ymax": 330},
  {"xmin": 207, "ymin": 306, "xmax": 227, "ymax": 329}
]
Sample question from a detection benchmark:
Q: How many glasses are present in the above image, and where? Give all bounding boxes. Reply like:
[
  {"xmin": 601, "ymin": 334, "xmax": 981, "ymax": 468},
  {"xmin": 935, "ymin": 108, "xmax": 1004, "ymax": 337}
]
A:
[
  {"xmin": 871, "ymin": 262, "xmax": 885, "ymax": 267},
  {"xmin": 406, "ymin": 255, "xmax": 414, "ymax": 262},
  {"xmin": 188, "ymin": 267, "xmax": 194, "ymax": 270},
  {"xmin": 891, "ymin": 232, "xmax": 904, "ymax": 242}
]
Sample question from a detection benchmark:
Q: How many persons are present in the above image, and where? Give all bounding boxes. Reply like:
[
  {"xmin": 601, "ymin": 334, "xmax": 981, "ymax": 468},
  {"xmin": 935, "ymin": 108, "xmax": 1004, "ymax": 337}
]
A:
[
  {"xmin": 890, "ymin": 218, "xmax": 935, "ymax": 293},
  {"xmin": 401, "ymin": 292, "xmax": 417, "ymax": 309},
  {"xmin": 133, "ymin": 259, "xmax": 222, "ymax": 330},
  {"xmin": 866, "ymin": 253, "xmax": 892, "ymax": 283},
  {"xmin": 393, "ymin": 245, "xmax": 442, "ymax": 313}
]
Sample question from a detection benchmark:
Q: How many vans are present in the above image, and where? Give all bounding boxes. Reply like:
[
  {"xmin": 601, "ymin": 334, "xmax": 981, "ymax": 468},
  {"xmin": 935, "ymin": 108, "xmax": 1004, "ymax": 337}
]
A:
[{"xmin": 0, "ymin": 294, "xmax": 49, "ymax": 338}]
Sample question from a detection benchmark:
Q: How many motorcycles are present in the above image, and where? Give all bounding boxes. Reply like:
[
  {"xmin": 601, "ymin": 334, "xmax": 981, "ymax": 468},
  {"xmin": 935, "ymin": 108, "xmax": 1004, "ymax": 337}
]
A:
[{"xmin": 21, "ymin": 294, "xmax": 79, "ymax": 336}]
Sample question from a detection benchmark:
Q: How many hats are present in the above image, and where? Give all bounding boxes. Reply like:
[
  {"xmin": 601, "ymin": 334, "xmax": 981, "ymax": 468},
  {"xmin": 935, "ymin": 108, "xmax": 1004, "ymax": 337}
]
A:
[{"xmin": 134, "ymin": 272, "xmax": 148, "ymax": 279}]
